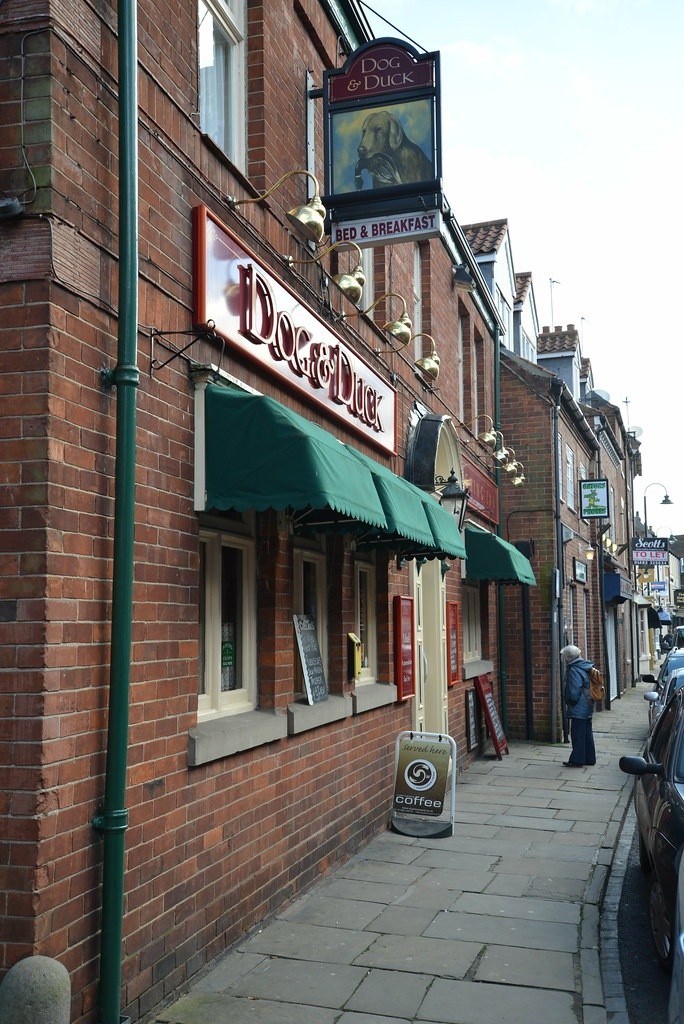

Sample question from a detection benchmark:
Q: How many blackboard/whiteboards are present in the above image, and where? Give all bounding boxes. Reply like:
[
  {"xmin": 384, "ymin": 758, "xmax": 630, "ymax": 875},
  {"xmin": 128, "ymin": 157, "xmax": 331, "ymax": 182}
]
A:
[
  {"xmin": 475, "ymin": 674, "xmax": 508, "ymax": 751},
  {"xmin": 293, "ymin": 614, "xmax": 329, "ymax": 705}
]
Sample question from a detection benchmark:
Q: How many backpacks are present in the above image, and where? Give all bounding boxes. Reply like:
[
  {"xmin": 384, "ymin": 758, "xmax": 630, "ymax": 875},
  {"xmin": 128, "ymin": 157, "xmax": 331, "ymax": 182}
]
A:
[{"xmin": 577, "ymin": 667, "xmax": 605, "ymax": 706}]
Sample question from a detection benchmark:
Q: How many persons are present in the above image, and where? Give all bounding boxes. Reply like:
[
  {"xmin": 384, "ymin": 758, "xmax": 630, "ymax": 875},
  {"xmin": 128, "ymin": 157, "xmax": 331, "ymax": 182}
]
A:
[
  {"xmin": 662, "ymin": 629, "xmax": 673, "ymax": 647},
  {"xmin": 560, "ymin": 645, "xmax": 596, "ymax": 767}
]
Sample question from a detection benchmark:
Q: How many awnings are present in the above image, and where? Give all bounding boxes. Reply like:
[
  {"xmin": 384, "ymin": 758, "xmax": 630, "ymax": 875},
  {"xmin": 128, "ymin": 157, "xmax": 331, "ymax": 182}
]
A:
[
  {"xmin": 658, "ymin": 611, "xmax": 672, "ymax": 625},
  {"xmin": 466, "ymin": 528, "xmax": 537, "ymax": 586},
  {"xmin": 204, "ymin": 385, "xmax": 468, "ymax": 562},
  {"xmin": 648, "ymin": 607, "xmax": 662, "ymax": 629},
  {"xmin": 605, "ymin": 573, "xmax": 633, "ymax": 605}
]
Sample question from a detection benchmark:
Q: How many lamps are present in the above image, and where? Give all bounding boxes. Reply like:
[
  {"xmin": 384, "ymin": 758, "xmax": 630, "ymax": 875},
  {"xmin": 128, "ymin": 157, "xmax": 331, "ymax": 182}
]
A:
[
  {"xmin": 373, "ymin": 333, "xmax": 440, "ymax": 383},
  {"xmin": 413, "ymin": 467, "xmax": 471, "ymax": 534},
  {"xmin": 486, "ymin": 461, "xmax": 526, "ymax": 490},
  {"xmin": 228, "ymin": 169, "xmax": 326, "ymax": 245},
  {"xmin": 339, "ymin": 293, "xmax": 412, "ymax": 346},
  {"xmin": 577, "ymin": 540, "xmax": 595, "ymax": 561},
  {"xmin": 643, "ymin": 568, "xmax": 650, "ymax": 579},
  {"xmin": 282, "ymin": 239, "xmax": 365, "ymax": 306},
  {"xmin": 455, "ymin": 414, "xmax": 497, "ymax": 449},
  {"xmin": 478, "ymin": 447, "xmax": 518, "ymax": 478},
  {"xmin": 451, "ymin": 263, "xmax": 477, "ymax": 292},
  {"xmin": 464, "ymin": 430, "xmax": 510, "ymax": 467}
]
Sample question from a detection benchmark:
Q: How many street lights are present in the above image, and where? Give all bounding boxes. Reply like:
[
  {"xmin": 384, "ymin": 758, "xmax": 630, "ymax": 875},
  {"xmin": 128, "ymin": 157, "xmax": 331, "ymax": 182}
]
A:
[{"xmin": 642, "ymin": 483, "xmax": 673, "ymax": 545}]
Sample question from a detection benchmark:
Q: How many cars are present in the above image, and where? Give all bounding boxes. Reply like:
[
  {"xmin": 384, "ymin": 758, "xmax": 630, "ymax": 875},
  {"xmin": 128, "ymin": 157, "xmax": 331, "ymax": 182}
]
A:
[
  {"xmin": 642, "ymin": 667, "xmax": 684, "ymax": 731},
  {"xmin": 641, "ymin": 626, "xmax": 684, "ymax": 700},
  {"xmin": 618, "ymin": 685, "xmax": 684, "ymax": 971}
]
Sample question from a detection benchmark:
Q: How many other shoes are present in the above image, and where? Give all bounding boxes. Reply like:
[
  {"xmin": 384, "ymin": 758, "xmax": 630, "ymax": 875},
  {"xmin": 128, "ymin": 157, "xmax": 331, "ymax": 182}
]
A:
[
  {"xmin": 583, "ymin": 761, "xmax": 596, "ymax": 765},
  {"xmin": 563, "ymin": 761, "xmax": 584, "ymax": 767}
]
